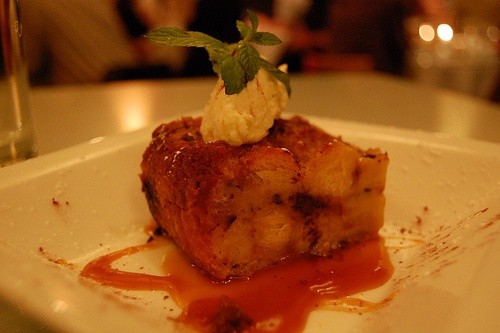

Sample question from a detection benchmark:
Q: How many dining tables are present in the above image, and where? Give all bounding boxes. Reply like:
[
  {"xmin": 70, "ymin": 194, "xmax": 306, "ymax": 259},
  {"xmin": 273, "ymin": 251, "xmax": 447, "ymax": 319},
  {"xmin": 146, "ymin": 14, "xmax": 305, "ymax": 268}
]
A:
[{"xmin": 0, "ymin": 70, "xmax": 500, "ymax": 333}]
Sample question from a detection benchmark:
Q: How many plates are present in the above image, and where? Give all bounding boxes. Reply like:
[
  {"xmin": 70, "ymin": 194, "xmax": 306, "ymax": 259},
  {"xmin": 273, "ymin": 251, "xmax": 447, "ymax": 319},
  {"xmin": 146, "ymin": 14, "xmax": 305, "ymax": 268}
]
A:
[{"xmin": 2, "ymin": 101, "xmax": 499, "ymax": 333}]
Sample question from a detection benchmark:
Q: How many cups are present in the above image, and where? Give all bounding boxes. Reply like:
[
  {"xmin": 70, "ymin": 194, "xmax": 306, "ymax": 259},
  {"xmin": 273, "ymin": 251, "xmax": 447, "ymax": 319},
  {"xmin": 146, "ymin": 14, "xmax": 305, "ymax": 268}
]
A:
[{"xmin": 0, "ymin": 0, "xmax": 38, "ymax": 164}]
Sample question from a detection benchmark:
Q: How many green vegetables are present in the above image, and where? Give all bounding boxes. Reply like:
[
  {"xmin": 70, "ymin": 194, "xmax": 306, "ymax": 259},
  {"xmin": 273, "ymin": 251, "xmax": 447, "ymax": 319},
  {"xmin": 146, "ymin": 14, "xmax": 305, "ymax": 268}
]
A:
[{"xmin": 143, "ymin": 8, "xmax": 292, "ymax": 98}]
